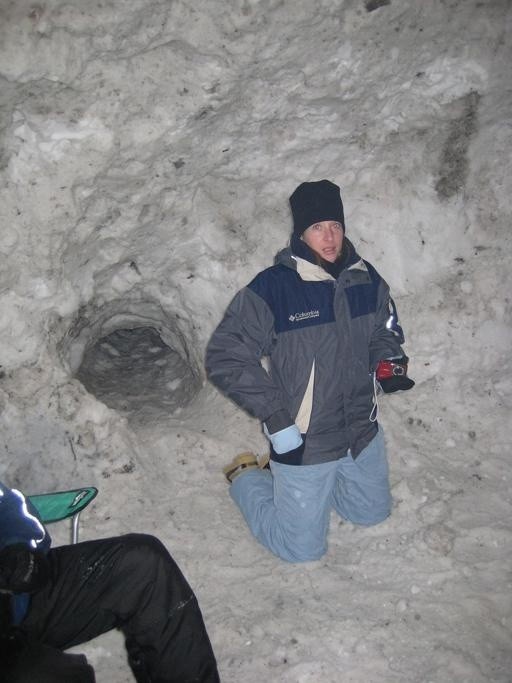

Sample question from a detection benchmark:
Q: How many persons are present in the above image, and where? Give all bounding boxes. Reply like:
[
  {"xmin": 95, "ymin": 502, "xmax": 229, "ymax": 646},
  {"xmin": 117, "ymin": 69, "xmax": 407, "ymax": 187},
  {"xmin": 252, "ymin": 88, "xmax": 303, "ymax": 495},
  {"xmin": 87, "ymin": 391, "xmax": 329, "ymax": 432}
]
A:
[
  {"xmin": 205, "ymin": 178, "xmax": 413, "ymax": 562},
  {"xmin": 0, "ymin": 472, "xmax": 220, "ymax": 683}
]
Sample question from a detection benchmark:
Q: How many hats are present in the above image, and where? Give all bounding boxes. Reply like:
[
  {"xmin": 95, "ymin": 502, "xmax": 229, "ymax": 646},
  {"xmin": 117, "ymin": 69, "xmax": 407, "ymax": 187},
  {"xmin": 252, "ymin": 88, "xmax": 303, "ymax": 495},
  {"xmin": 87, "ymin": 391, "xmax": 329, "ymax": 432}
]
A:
[{"xmin": 289, "ymin": 179, "xmax": 345, "ymax": 238}]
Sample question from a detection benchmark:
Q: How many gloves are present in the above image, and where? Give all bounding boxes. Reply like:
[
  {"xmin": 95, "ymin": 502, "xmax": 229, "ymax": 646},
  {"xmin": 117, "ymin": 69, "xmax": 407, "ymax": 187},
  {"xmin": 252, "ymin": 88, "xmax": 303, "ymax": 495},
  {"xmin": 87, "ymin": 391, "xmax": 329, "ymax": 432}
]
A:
[{"xmin": 262, "ymin": 409, "xmax": 304, "ymax": 455}]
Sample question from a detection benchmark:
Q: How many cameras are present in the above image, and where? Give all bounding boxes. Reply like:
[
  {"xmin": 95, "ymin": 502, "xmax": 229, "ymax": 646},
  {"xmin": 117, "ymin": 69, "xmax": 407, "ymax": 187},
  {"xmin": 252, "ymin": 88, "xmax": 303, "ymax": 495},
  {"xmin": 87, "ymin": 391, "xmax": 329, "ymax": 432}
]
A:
[{"xmin": 376, "ymin": 359, "xmax": 407, "ymax": 381}]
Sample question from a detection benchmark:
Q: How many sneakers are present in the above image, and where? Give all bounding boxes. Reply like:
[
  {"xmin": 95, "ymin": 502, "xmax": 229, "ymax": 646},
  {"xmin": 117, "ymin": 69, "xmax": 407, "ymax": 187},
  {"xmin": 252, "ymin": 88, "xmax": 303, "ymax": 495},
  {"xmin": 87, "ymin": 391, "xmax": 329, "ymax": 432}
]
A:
[{"xmin": 223, "ymin": 452, "xmax": 270, "ymax": 483}]
